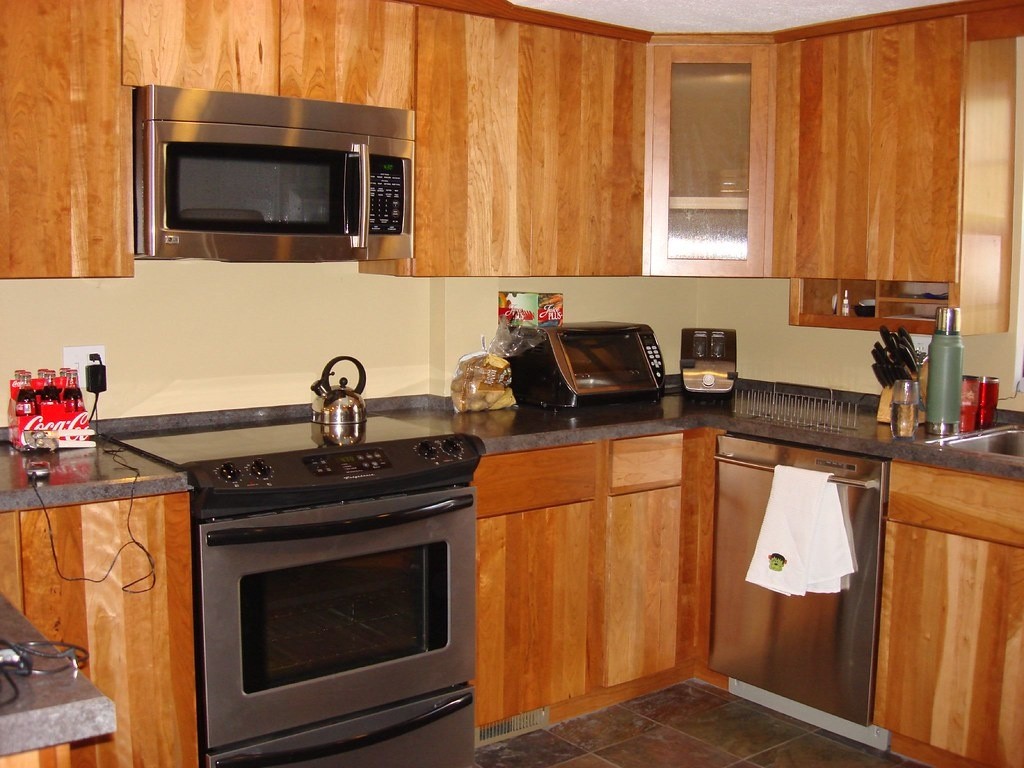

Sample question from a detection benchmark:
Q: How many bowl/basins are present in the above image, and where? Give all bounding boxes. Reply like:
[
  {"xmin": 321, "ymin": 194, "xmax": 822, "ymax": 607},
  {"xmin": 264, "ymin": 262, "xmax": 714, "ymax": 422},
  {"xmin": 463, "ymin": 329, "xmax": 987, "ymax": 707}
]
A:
[
  {"xmin": 720, "ymin": 168, "xmax": 748, "ymax": 197},
  {"xmin": 854, "ymin": 299, "xmax": 875, "ymax": 317}
]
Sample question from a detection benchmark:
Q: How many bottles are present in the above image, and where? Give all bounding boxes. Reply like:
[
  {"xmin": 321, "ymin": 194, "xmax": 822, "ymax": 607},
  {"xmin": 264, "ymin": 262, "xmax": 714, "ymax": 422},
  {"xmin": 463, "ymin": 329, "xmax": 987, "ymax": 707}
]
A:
[
  {"xmin": 926, "ymin": 306, "xmax": 964, "ymax": 435},
  {"xmin": 842, "ymin": 290, "xmax": 850, "ymax": 316},
  {"xmin": 14, "ymin": 368, "xmax": 84, "ymax": 415}
]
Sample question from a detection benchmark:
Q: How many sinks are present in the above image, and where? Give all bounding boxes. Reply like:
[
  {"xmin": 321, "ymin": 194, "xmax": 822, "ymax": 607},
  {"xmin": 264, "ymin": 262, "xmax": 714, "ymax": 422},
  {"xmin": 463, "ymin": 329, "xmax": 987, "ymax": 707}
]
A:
[{"xmin": 943, "ymin": 428, "xmax": 1024, "ymax": 459}]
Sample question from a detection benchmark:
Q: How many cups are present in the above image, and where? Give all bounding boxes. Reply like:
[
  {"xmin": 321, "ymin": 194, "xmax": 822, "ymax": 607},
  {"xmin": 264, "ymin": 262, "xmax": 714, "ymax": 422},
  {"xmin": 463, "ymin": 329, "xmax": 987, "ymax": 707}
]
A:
[
  {"xmin": 978, "ymin": 376, "xmax": 1000, "ymax": 429},
  {"xmin": 959, "ymin": 375, "xmax": 980, "ymax": 432},
  {"xmin": 891, "ymin": 380, "xmax": 920, "ymax": 442}
]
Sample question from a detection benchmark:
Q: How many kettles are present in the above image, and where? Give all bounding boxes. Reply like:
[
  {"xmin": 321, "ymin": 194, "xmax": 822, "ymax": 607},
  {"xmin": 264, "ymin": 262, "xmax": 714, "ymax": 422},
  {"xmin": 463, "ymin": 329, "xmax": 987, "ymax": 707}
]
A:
[{"xmin": 310, "ymin": 356, "xmax": 366, "ymax": 424}]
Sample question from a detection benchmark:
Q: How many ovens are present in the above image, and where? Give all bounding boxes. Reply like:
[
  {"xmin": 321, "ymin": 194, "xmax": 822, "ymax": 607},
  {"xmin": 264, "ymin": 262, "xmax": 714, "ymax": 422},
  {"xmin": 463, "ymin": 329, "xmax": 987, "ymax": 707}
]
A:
[
  {"xmin": 190, "ymin": 485, "xmax": 477, "ymax": 768},
  {"xmin": 501, "ymin": 321, "xmax": 665, "ymax": 414}
]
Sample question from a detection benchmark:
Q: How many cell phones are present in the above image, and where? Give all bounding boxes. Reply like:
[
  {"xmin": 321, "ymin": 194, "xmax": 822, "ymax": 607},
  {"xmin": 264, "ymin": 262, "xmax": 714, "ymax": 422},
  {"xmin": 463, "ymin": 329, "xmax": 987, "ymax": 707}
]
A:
[{"xmin": 26, "ymin": 461, "xmax": 51, "ymax": 476}]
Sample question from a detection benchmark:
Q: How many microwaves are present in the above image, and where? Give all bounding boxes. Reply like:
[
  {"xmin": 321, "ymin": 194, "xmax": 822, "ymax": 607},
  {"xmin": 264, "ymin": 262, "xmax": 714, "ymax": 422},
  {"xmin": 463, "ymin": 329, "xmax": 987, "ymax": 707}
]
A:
[{"xmin": 136, "ymin": 84, "xmax": 415, "ymax": 263}]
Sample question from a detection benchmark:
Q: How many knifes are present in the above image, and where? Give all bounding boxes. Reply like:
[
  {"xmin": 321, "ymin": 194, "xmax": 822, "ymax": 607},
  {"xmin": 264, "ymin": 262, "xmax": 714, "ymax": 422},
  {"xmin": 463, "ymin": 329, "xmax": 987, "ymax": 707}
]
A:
[{"xmin": 870, "ymin": 325, "xmax": 918, "ymax": 388}]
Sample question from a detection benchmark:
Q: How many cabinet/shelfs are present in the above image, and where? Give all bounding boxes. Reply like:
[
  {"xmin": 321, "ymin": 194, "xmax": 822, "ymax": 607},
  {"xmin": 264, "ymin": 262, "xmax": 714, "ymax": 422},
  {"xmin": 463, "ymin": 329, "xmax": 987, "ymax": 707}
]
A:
[
  {"xmin": 0, "ymin": 742, "xmax": 73, "ymax": 768},
  {"xmin": 876, "ymin": 463, "xmax": 1024, "ymax": 768},
  {"xmin": 0, "ymin": 0, "xmax": 1024, "ymax": 337},
  {"xmin": 474, "ymin": 425, "xmax": 704, "ymax": 747},
  {"xmin": 0, "ymin": 486, "xmax": 196, "ymax": 768}
]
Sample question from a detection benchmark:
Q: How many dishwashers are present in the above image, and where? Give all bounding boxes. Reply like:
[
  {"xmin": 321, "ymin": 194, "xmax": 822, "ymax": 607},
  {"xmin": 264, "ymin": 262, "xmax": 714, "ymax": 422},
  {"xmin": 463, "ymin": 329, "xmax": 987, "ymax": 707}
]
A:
[{"xmin": 707, "ymin": 434, "xmax": 890, "ymax": 728}]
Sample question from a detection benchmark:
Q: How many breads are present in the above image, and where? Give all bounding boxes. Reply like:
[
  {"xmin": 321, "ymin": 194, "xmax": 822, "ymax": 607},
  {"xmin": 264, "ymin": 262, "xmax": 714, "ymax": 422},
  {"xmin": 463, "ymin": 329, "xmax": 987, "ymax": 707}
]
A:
[{"xmin": 450, "ymin": 357, "xmax": 514, "ymax": 412}]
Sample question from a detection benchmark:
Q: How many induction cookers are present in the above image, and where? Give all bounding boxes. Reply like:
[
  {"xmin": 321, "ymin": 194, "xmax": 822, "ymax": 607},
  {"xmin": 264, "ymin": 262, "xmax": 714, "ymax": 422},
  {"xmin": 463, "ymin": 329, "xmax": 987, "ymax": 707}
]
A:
[{"xmin": 100, "ymin": 412, "xmax": 480, "ymax": 519}]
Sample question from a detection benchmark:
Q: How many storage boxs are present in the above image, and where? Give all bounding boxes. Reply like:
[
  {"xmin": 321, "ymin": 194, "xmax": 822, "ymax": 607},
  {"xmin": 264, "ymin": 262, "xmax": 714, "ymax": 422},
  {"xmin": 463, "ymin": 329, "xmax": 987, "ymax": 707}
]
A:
[
  {"xmin": 498, "ymin": 290, "xmax": 563, "ymax": 327},
  {"xmin": 7, "ymin": 377, "xmax": 90, "ymax": 447}
]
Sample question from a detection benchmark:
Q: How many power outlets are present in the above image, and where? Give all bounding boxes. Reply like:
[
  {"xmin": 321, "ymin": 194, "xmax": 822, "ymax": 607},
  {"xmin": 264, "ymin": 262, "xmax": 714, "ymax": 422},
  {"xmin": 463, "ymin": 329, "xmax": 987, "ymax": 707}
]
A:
[{"xmin": 63, "ymin": 346, "xmax": 105, "ymax": 387}]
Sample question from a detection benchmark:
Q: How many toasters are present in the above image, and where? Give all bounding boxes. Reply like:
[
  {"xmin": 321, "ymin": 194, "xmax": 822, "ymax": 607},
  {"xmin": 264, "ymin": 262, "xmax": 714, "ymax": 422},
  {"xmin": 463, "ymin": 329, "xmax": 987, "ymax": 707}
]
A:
[{"xmin": 681, "ymin": 328, "xmax": 737, "ymax": 395}]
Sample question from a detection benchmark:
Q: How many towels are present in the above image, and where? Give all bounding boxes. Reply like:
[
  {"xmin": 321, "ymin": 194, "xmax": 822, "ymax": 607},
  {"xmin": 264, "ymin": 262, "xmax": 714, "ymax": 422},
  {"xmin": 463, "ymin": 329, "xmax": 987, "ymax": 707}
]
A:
[{"xmin": 744, "ymin": 463, "xmax": 859, "ymax": 598}]
